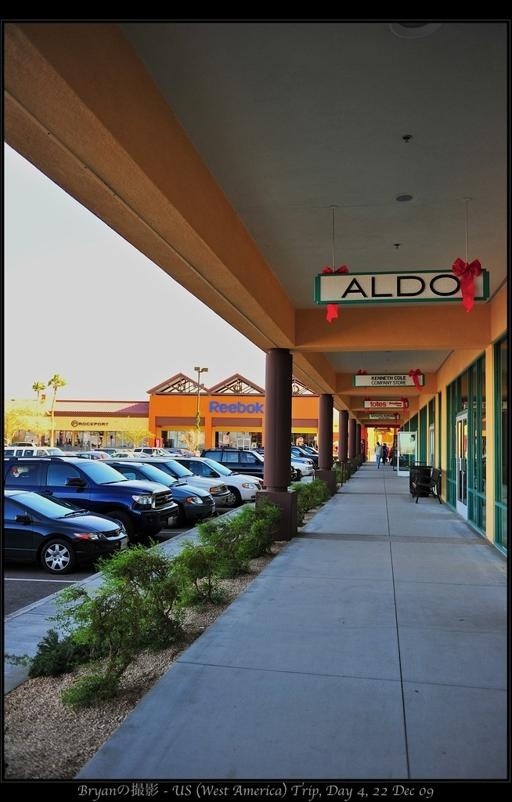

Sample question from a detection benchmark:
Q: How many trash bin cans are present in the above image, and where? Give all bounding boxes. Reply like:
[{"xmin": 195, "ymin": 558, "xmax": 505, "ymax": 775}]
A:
[{"xmin": 410, "ymin": 466, "xmax": 431, "ymax": 497}]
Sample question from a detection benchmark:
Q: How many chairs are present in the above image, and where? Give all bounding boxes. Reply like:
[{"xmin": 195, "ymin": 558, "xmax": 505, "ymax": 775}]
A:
[{"xmin": 411, "ymin": 468, "xmax": 442, "ymax": 504}]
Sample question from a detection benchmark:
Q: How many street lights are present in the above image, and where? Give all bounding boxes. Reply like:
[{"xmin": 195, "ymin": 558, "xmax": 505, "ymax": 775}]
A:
[{"xmin": 194, "ymin": 367, "xmax": 208, "ymax": 456}]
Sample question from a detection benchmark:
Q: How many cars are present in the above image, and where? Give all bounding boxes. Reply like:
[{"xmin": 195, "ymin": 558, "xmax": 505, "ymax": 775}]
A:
[
  {"xmin": 4, "ymin": 442, "xmax": 201, "ymax": 461},
  {"xmin": 163, "ymin": 454, "xmax": 264, "ymax": 501},
  {"xmin": 459, "ymin": 469, "xmax": 484, "ymax": 499},
  {"xmin": 95, "ymin": 458, "xmax": 231, "ymax": 507},
  {"xmin": 470, "ymin": 454, "xmax": 486, "ymax": 480},
  {"xmin": 247, "ymin": 445, "xmax": 338, "ymax": 482},
  {"xmin": 3, "ymin": 488, "xmax": 129, "ymax": 575},
  {"xmin": 96, "ymin": 461, "xmax": 216, "ymax": 530}
]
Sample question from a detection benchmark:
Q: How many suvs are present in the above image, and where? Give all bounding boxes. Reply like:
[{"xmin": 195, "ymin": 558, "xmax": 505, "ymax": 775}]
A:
[
  {"xmin": 2, "ymin": 456, "xmax": 180, "ymax": 548},
  {"xmin": 200, "ymin": 447, "xmax": 297, "ymax": 486}
]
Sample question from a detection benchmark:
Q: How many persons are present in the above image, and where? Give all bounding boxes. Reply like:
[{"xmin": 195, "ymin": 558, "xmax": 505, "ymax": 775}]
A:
[
  {"xmin": 408, "ymin": 435, "xmax": 415, "ymax": 467},
  {"xmin": 381, "ymin": 443, "xmax": 386, "ymax": 463},
  {"xmin": 374, "ymin": 442, "xmax": 384, "ymax": 469},
  {"xmin": 385, "ymin": 446, "xmax": 389, "ymax": 462}
]
